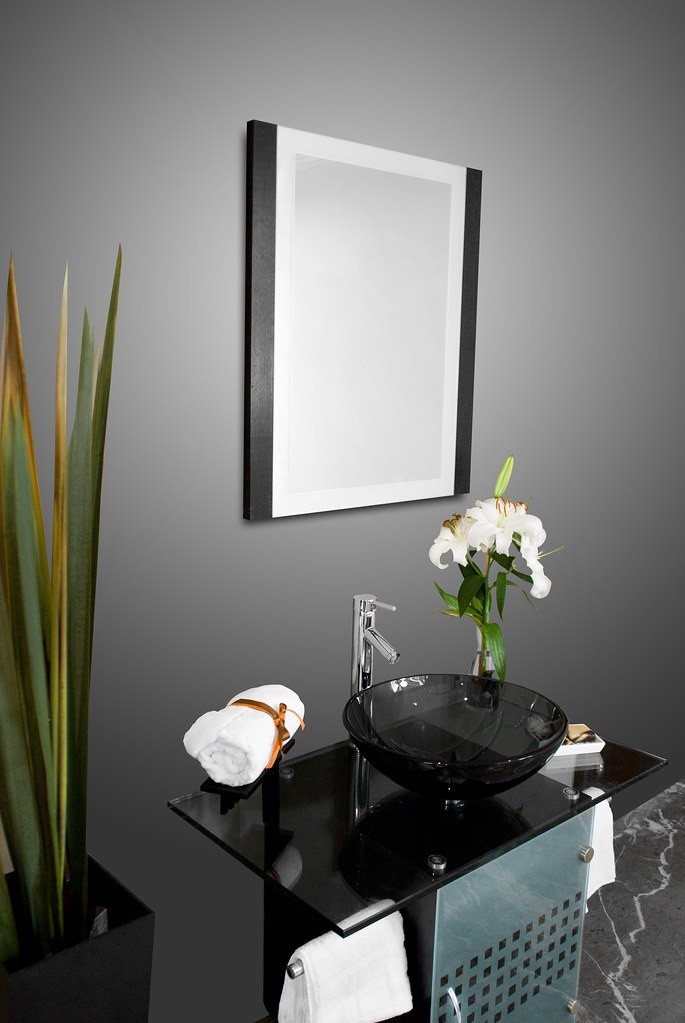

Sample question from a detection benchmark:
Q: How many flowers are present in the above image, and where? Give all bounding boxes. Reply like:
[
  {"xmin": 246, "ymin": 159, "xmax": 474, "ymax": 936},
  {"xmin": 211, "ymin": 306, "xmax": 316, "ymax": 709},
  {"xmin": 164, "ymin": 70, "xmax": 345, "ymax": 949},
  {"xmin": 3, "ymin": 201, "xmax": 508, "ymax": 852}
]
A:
[{"xmin": 428, "ymin": 453, "xmax": 564, "ymax": 681}]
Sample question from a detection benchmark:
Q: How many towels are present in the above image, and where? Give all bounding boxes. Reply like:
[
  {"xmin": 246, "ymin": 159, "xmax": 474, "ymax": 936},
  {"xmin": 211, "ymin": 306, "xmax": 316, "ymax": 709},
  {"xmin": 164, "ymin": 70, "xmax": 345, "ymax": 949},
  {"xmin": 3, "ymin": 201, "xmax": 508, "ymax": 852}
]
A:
[
  {"xmin": 275, "ymin": 898, "xmax": 415, "ymax": 1023},
  {"xmin": 181, "ymin": 682, "xmax": 307, "ymax": 787},
  {"xmin": 580, "ymin": 786, "xmax": 618, "ymax": 915}
]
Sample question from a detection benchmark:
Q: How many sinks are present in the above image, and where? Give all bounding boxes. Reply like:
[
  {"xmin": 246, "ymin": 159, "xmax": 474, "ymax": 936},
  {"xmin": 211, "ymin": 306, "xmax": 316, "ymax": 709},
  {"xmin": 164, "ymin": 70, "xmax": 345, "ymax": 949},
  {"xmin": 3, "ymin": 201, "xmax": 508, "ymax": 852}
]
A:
[{"xmin": 341, "ymin": 672, "xmax": 571, "ymax": 802}]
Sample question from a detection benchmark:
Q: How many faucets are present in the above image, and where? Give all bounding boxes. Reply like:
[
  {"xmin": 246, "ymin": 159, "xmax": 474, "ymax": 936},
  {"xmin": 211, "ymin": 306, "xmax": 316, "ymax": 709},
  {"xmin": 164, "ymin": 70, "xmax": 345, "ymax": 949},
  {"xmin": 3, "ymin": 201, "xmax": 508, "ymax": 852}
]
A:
[
  {"xmin": 349, "ymin": 742, "xmax": 383, "ymax": 845},
  {"xmin": 349, "ymin": 591, "xmax": 402, "ymax": 700}
]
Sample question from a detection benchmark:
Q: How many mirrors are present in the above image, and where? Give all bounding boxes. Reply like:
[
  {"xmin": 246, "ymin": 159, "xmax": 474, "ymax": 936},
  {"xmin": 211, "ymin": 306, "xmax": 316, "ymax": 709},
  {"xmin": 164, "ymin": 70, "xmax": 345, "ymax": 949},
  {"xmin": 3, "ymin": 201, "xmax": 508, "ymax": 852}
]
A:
[{"xmin": 241, "ymin": 118, "xmax": 483, "ymax": 524}]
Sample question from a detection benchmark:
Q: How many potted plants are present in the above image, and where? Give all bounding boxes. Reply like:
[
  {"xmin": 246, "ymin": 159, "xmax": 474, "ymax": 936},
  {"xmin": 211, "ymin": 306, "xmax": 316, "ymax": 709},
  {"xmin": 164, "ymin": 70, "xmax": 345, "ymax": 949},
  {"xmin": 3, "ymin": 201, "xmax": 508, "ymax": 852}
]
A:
[{"xmin": 0, "ymin": 245, "xmax": 159, "ymax": 1023}]
